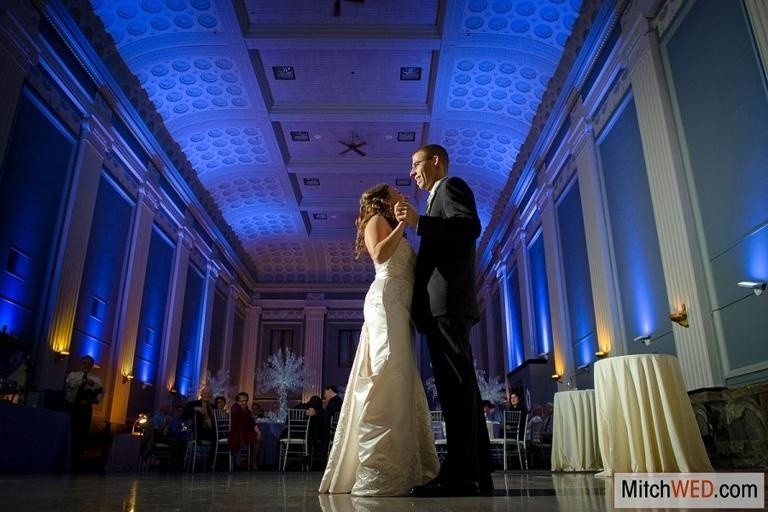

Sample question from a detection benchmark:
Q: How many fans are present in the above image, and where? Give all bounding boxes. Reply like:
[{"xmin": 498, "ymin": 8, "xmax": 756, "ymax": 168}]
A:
[
  {"xmin": 331, "ymin": 0, "xmax": 366, "ymax": 17},
  {"xmin": 338, "ymin": 134, "xmax": 367, "ymax": 156}
]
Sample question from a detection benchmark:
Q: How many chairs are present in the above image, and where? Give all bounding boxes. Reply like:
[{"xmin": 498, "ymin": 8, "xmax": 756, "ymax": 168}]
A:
[{"xmin": 136, "ymin": 407, "xmax": 548, "ymax": 479}]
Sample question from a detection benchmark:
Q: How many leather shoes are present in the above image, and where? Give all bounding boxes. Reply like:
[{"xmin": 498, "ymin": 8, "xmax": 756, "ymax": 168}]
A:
[{"xmin": 409, "ymin": 473, "xmax": 495, "ymax": 497}]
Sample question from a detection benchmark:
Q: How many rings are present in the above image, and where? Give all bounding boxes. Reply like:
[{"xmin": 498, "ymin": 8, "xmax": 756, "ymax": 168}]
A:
[{"xmin": 399, "ymin": 210, "xmax": 401, "ymax": 215}]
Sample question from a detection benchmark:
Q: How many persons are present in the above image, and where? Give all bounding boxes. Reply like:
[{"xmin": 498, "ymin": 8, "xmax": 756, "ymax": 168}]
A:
[
  {"xmin": 152, "ymin": 384, "xmax": 344, "ymax": 470},
  {"xmin": 523, "ymin": 402, "xmax": 553, "ymax": 470},
  {"xmin": 392, "ymin": 144, "xmax": 497, "ymax": 496},
  {"xmin": 64, "ymin": 355, "xmax": 104, "ymax": 435},
  {"xmin": 482, "ymin": 400, "xmax": 503, "ymax": 438},
  {"xmin": 317, "ymin": 183, "xmax": 440, "ymax": 498},
  {"xmin": 499, "ymin": 391, "xmax": 528, "ymax": 441},
  {"xmin": 526, "ymin": 402, "xmax": 543, "ymax": 443}
]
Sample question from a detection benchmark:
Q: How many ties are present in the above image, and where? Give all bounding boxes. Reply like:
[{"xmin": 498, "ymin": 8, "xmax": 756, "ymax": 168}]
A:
[{"xmin": 73, "ymin": 373, "xmax": 88, "ymax": 404}]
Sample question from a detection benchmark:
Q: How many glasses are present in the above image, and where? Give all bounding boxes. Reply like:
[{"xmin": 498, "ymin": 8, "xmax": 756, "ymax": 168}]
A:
[{"xmin": 410, "ymin": 157, "xmax": 433, "ymax": 170}]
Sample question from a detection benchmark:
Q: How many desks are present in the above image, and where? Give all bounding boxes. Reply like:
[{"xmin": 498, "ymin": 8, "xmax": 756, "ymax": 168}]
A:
[
  {"xmin": 550, "ymin": 390, "xmax": 602, "ymax": 473},
  {"xmin": 594, "ymin": 353, "xmax": 717, "ymax": 476}
]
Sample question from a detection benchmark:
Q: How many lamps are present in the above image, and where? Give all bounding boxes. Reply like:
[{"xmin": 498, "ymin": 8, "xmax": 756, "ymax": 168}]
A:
[
  {"xmin": 55, "ymin": 351, "xmax": 70, "ymax": 361},
  {"xmin": 595, "ymin": 351, "xmax": 606, "ymax": 359},
  {"xmin": 123, "ymin": 375, "xmax": 134, "ymax": 383},
  {"xmin": 132, "ymin": 414, "xmax": 148, "ymax": 435},
  {"xmin": 552, "ymin": 374, "xmax": 563, "ymax": 384},
  {"xmin": 670, "ymin": 304, "xmax": 689, "ymax": 328}
]
